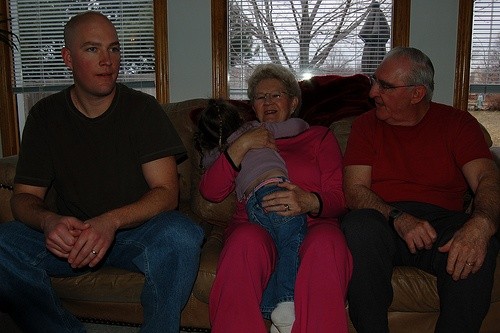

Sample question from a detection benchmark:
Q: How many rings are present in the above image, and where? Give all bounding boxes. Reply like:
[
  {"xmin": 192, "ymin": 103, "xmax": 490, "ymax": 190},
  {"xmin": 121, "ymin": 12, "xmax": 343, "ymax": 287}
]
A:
[
  {"xmin": 467, "ymin": 261, "xmax": 476, "ymax": 266},
  {"xmin": 91, "ymin": 250, "xmax": 97, "ymax": 255},
  {"xmin": 284, "ymin": 204, "xmax": 289, "ymax": 211}
]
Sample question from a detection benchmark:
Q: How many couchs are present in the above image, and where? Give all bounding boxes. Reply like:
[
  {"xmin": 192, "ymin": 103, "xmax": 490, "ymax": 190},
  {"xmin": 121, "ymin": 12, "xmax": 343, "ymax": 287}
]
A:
[{"xmin": 0, "ymin": 100, "xmax": 500, "ymax": 333}]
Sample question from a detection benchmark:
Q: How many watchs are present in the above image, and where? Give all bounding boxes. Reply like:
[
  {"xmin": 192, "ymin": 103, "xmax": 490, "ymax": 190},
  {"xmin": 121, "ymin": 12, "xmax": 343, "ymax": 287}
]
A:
[{"xmin": 388, "ymin": 208, "xmax": 405, "ymax": 230}]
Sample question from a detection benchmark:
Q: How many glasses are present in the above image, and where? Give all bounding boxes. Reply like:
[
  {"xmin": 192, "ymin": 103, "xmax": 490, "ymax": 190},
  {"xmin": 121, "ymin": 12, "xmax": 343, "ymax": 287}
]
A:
[
  {"xmin": 254, "ymin": 91, "xmax": 287, "ymax": 102},
  {"xmin": 367, "ymin": 72, "xmax": 420, "ymax": 92}
]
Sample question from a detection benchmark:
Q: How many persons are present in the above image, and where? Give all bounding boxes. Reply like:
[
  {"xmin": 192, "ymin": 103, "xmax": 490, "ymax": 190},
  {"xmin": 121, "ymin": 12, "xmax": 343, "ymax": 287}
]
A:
[
  {"xmin": 192, "ymin": 98, "xmax": 309, "ymax": 333},
  {"xmin": 0, "ymin": 11, "xmax": 207, "ymax": 333},
  {"xmin": 200, "ymin": 63, "xmax": 354, "ymax": 333},
  {"xmin": 342, "ymin": 46, "xmax": 500, "ymax": 333}
]
